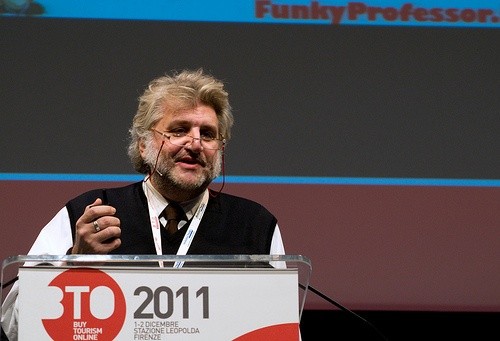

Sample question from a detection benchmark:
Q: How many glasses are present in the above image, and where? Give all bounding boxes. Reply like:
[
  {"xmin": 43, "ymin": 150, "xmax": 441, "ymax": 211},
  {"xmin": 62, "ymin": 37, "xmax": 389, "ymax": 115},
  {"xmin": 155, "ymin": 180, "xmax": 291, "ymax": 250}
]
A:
[{"xmin": 152, "ymin": 128, "xmax": 225, "ymax": 149}]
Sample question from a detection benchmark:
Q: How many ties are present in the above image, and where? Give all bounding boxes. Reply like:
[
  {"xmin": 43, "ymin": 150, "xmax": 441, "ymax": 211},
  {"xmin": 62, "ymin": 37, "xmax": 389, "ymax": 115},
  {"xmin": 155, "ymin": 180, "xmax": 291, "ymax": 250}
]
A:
[{"xmin": 163, "ymin": 205, "xmax": 183, "ymax": 233}]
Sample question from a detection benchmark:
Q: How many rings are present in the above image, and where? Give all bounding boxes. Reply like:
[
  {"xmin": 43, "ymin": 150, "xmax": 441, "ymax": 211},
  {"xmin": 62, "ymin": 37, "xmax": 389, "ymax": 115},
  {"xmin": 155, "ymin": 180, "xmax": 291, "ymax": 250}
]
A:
[{"xmin": 93, "ymin": 220, "xmax": 101, "ymax": 233}]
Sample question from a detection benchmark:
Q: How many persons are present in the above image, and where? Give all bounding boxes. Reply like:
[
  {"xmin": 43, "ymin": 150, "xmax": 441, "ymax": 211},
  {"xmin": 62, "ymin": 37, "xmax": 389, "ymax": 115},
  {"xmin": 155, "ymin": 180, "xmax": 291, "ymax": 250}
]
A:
[{"xmin": 1, "ymin": 69, "xmax": 289, "ymax": 341}]
